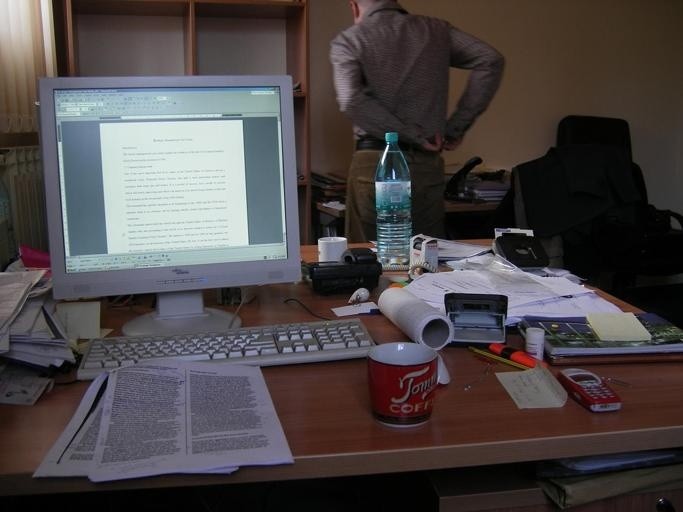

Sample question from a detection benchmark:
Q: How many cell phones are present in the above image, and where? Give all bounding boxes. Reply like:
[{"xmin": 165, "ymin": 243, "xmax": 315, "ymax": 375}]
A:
[{"xmin": 557, "ymin": 368, "xmax": 623, "ymax": 412}]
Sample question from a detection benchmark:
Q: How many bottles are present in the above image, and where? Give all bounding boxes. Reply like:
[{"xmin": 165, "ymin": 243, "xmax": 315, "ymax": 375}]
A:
[
  {"xmin": 375, "ymin": 131, "xmax": 412, "ymax": 270},
  {"xmin": 525, "ymin": 326, "xmax": 544, "ymax": 363}
]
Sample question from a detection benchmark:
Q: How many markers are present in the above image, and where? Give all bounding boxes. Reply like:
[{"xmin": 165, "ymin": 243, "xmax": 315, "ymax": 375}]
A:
[{"xmin": 489, "ymin": 345, "xmax": 541, "ymax": 367}]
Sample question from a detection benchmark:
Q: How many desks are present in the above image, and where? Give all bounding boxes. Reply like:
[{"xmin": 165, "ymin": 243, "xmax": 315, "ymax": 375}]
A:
[
  {"xmin": 0, "ymin": 239, "xmax": 683, "ymax": 499},
  {"xmin": 315, "ymin": 190, "xmax": 506, "ymax": 232}
]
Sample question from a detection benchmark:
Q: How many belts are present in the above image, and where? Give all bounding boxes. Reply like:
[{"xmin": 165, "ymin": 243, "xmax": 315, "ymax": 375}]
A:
[{"xmin": 353, "ymin": 137, "xmax": 421, "ymax": 153}]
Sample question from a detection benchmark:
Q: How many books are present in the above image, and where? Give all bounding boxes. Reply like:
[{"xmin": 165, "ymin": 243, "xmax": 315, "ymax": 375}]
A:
[
  {"xmin": 521, "ymin": 310, "xmax": 683, "ymax": 356},
  {"xmin": 516, "ymin": 318, "xmax": 683, "ymax": 366},
  {"xmin": 309, "ymin": 167, "xmax": 351, "ymax": 207},
  {"xmin": 443, "ymin": 161, "xmax": 514, "ymax": 204},
  {"xmin": 0, "ymin": 244, "xmax": 116, "ymax": 371},
  {"xmin": 370, "ymin": 232, "xmax": 494, "ymax": 262}
]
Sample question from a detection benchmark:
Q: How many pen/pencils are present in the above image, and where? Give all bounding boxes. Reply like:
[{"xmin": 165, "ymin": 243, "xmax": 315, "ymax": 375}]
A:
[{"xmin": 467, "ymin": 347, "xmax": 532, "ymax": 370}]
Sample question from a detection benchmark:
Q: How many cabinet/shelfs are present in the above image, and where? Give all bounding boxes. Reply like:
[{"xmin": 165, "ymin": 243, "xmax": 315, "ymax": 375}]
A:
[{"xmin": 64, "ymin": 1, "xmax": 313, "ymax": 254}]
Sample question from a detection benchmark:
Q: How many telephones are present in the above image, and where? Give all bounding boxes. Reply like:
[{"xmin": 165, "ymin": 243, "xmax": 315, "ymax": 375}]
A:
[{"xmin": 308, "ymin": 247, "xmax": 382, "ymax": 294}]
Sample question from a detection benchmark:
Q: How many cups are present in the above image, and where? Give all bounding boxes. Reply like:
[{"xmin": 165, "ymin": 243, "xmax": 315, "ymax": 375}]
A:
[
  {"xmin": 318, "ymin": 235, "xmax": 347, "ymax": 265},
  {"xmin": 366, "ymin": 343, "xmax": 443, "ymax": 429}
]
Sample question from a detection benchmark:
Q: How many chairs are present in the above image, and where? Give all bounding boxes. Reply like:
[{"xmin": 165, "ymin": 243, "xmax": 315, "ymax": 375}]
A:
[{"xmin": 510, "ymin": 104, "xmax": 683, "ymax": 318}]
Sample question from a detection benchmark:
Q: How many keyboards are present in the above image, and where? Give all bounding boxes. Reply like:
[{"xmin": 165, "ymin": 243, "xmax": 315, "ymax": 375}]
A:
[{"xmin": 76, "ymin": 317, "xmax": 377, "ymax": 381}]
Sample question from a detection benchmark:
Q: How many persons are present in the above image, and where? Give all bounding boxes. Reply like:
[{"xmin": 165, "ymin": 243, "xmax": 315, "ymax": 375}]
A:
[{"xmin": 329, "ymin": 0, "xmax": 507, "ymax": 243}]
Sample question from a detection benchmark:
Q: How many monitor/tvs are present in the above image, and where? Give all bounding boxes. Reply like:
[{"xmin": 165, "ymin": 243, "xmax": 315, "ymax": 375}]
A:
[{"xmin": 36, "ymin": 73, "xmax": 303, "ymax": 336}]
